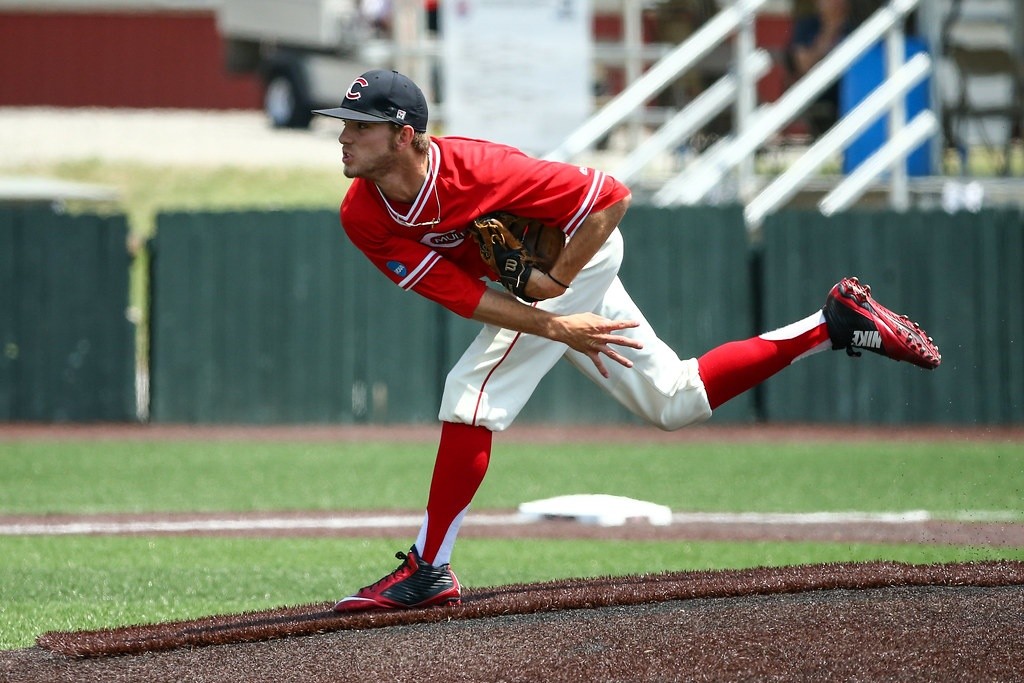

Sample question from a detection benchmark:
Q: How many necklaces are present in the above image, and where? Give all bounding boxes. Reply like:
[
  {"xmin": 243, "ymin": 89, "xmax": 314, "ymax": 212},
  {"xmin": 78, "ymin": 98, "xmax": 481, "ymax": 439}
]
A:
[{"xmin": 384, "ymin": 162, "xmax": 441, "ymax": 227}]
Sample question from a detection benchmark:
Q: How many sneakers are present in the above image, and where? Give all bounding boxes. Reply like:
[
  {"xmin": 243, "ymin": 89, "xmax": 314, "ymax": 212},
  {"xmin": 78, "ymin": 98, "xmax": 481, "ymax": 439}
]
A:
[
  {"xmin": 334, "ymin": 545, "xmax": 462, "ymax": 612},
  {"xmin": 822, "ymin": 277, "xmax": 942, "ymax": 370}
]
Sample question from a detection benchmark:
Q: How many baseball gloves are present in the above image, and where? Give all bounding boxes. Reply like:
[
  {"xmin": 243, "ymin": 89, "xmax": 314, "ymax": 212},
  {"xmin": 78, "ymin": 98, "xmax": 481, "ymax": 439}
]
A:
[{"xmin": 468, "ymin": 213, "xmax": 567, "ymax": 304}]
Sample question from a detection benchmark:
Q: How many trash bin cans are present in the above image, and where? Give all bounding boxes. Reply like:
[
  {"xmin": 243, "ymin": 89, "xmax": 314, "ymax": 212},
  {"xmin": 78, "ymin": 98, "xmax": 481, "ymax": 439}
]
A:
[{"xmin": 836, "ymin": 34, "xmax": 933, "ymax": 178}]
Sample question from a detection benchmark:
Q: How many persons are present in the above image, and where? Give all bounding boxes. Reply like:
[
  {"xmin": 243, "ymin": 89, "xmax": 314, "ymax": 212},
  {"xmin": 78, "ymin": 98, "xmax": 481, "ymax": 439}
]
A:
[
  {"xmin": 312, "ymin": 69, "xmax": 943, "ymax": 611},
  {"xmin": 786, "ymin": 0, "xmax": 860, "ymax": 138}
]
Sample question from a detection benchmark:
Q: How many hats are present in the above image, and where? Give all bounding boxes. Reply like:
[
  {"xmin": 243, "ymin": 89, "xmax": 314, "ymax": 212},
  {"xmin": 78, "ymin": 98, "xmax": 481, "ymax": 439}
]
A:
[{"xmin": 311, "ymin": 71, "xmax": 429, "ymax": 133}]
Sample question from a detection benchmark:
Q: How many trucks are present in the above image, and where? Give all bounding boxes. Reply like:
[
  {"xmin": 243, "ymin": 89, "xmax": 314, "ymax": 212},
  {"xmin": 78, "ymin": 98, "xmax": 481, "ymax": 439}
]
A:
[{"xmin": 213, "ymin": 0, "xmax": 446, "ymax": 133}]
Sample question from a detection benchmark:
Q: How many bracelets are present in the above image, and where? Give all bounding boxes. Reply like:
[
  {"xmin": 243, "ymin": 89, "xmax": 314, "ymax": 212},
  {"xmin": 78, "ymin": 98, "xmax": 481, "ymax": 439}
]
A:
[{"xmin": 548, "ymin": 272, "xmax": 569, "ymax": 288}]
